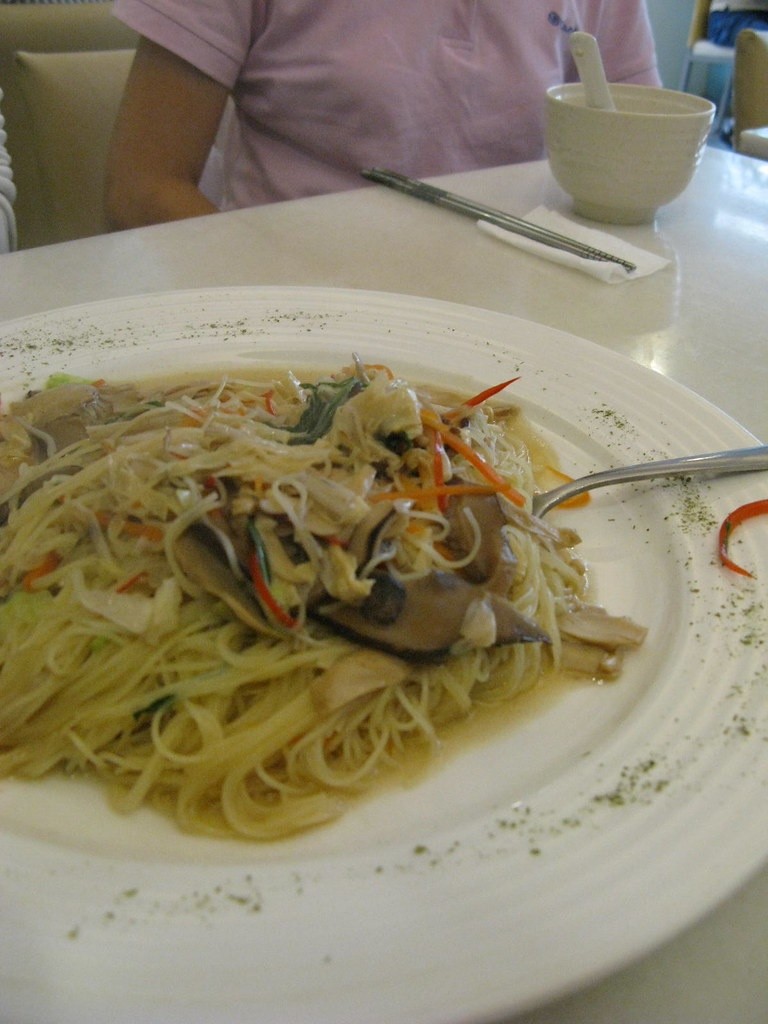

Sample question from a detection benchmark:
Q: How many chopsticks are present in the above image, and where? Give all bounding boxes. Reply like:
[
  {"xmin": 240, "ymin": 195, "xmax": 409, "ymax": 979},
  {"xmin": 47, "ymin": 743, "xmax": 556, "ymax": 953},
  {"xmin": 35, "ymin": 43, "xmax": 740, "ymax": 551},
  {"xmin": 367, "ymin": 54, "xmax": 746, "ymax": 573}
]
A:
[{"xmin": 360, "ymin": 167, "xmax": 636, "ymax": 273}]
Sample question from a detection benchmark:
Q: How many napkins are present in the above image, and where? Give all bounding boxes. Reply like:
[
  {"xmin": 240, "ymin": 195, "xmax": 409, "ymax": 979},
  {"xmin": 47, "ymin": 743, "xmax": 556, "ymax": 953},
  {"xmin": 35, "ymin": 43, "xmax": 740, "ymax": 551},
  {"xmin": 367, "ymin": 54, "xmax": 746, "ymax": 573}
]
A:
[{"xmin": 475, "ymin": 205, "xmax": 670, "ymax": 285}]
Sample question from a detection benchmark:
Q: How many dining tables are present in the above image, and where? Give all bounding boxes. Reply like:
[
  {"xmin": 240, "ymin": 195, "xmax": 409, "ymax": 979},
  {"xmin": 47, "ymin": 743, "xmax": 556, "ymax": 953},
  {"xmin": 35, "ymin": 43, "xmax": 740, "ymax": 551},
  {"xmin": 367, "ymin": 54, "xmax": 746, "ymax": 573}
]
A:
[{"xmin": 0, "ymin": 146, "xmax": 768, "ymax": 1023}]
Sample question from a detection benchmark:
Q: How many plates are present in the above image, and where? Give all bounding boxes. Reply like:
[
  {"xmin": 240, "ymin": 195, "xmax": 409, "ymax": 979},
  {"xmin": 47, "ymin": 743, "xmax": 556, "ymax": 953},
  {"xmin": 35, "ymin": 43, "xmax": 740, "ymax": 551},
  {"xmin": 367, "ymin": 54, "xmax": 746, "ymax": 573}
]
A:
[{"xmin": 0, "ymin": 286, "xmax": 767, "ymax": 1022}]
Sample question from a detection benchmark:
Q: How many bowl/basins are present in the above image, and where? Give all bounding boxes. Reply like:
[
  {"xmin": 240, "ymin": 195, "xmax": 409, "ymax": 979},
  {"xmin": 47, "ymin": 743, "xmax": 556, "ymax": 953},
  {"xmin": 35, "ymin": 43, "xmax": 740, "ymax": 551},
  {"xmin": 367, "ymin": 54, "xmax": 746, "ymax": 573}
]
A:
[{"xmin": 544, "ymin": 83, "xmax": 717, "ymax": 226}]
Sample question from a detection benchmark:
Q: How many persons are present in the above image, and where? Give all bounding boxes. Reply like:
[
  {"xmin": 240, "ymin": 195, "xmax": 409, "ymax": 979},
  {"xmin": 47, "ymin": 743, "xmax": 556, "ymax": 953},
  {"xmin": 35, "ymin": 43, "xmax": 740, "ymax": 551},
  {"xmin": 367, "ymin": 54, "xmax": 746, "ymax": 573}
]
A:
[
  {"xmin": 102, "ymin": 0, "xmax": 664, "ymax": 231},
  {"xmin": 1, "ymin": 86, "xmax": 18, "ymax": 258}
]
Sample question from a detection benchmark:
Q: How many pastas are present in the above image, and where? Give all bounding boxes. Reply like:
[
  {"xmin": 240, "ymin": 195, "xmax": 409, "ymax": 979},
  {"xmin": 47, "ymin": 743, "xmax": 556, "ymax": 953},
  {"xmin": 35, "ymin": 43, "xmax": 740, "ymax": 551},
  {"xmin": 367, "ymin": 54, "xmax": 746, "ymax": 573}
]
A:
[{"xmin": 0, "ymin": 372, "xmax": 587, "ymax": 843}]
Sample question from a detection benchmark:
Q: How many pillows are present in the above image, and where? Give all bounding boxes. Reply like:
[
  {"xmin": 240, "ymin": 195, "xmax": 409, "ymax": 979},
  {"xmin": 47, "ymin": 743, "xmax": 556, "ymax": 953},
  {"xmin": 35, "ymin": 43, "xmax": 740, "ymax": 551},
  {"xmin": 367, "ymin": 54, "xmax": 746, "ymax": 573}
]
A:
[{"xmin": 12, "ymin": 47, "xmax": 140, "ymax": 244}]
